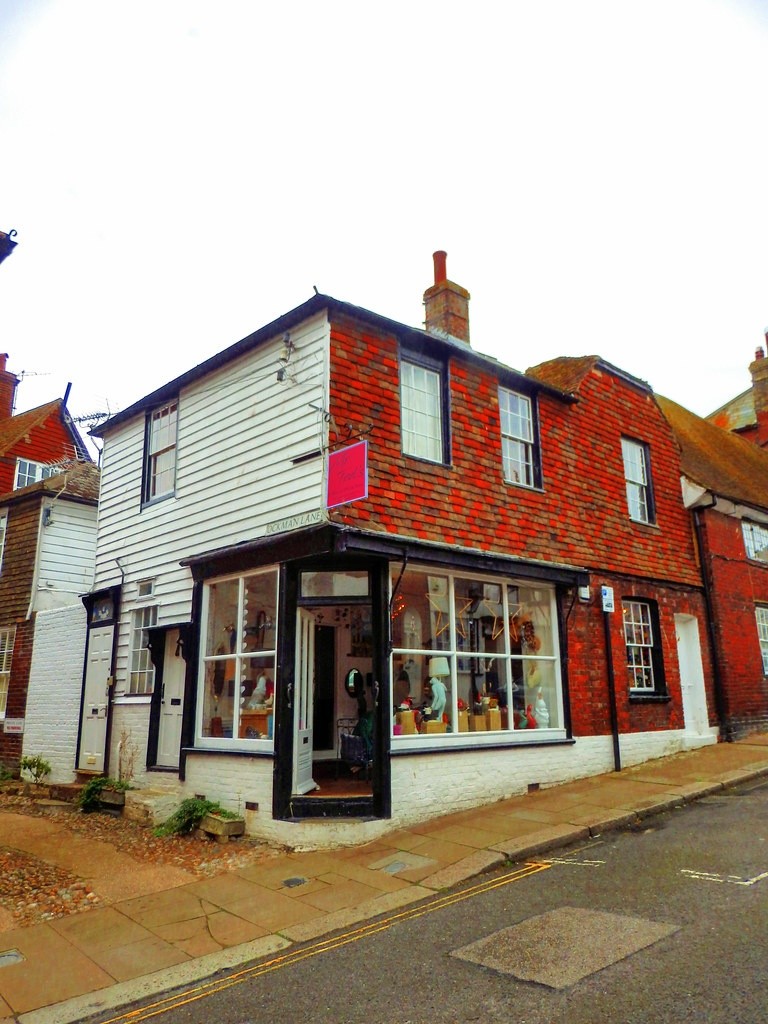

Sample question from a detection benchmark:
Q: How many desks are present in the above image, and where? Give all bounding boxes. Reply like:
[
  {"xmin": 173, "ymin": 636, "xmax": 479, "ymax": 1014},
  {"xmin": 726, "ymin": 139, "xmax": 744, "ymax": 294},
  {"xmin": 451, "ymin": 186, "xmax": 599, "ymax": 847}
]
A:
[{"xmin": 240, "ymin": 707, "xmax": 273, "ymax": 739}]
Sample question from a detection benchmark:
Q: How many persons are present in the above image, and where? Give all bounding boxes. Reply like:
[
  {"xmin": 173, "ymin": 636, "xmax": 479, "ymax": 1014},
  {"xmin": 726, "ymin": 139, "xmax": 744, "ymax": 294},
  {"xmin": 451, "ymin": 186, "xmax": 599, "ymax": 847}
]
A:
[
  {"xmin": 392, "ymin": 670, "xmax": 412, "ymax": 715},
  {"xmin": 423, "ymin": 676, "xmax": 449, "ymax": 722},
  {"xmin": 250, "ymin": 666, "xmax": 275, "ymax": 739}
]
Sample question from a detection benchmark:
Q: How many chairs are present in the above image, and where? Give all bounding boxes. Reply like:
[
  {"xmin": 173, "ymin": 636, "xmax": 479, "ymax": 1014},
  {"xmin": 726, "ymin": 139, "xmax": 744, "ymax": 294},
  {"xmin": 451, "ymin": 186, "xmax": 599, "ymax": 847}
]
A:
[{"xmin": 334, "ymin": 718, "xmax": 374, "ymax": 785}]
